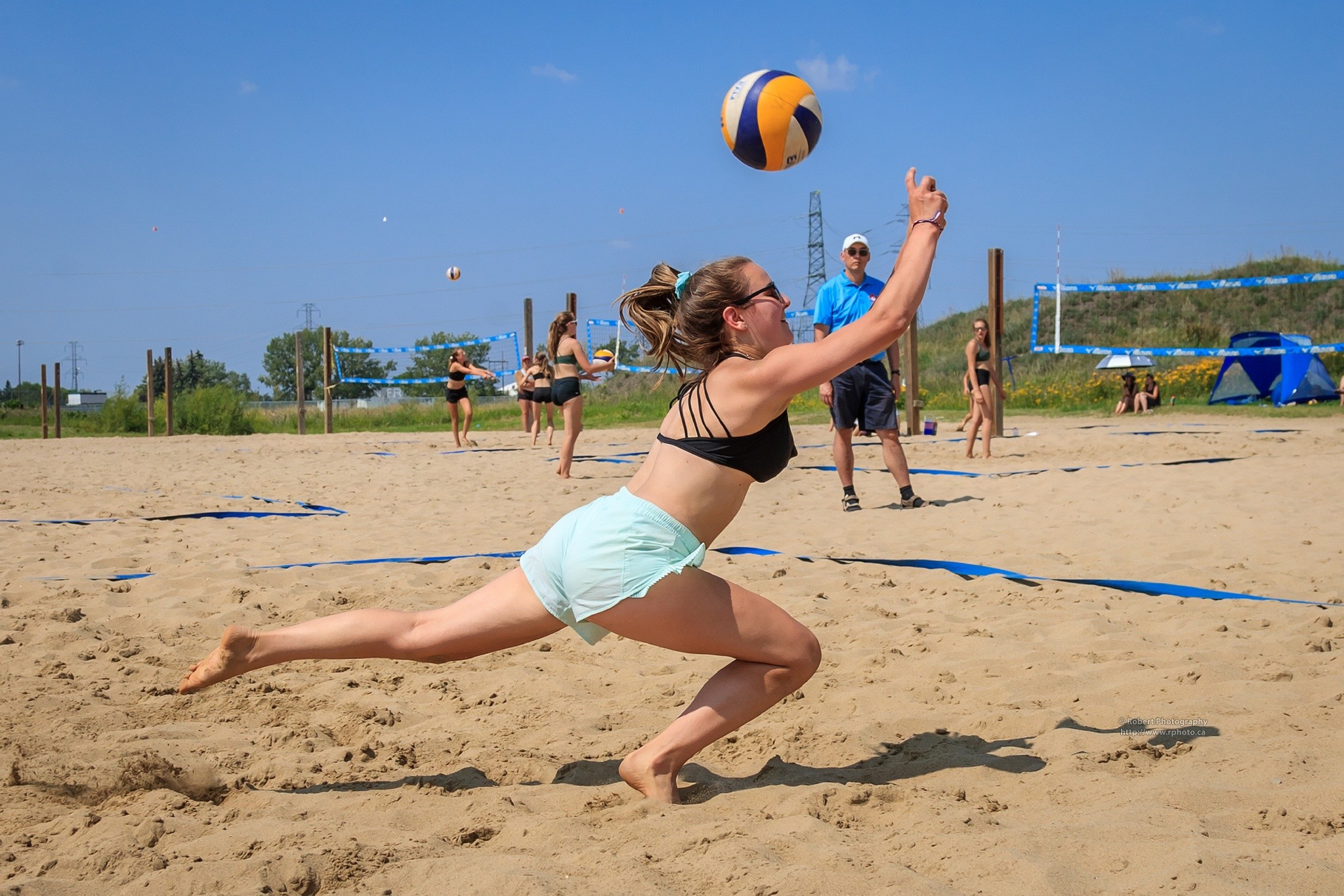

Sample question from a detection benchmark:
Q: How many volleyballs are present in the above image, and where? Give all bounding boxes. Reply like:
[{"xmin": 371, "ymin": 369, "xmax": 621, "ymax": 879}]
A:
[
  {"xmin": 446, "ymin": 266, "xmax": 461, "ymax": 281},
  {"xmin": 593, "ymin": 348, "xmax": 614, "ymax": 372},
  {"xmin": 719, "ymin": 68, "xmax": 822, "ymax": 171}
]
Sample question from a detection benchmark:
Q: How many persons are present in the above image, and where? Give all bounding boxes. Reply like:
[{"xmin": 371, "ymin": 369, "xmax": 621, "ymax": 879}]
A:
[
  {"xmin": 515, "ymin": 356, "xmax": 534, "ymax": 433},
  {"xmin": 179, "ymin": 168, "xmax": 950, "ymax": 809},
  {"xmin": 546, "ymin": 311, "xmax": 618, "ymax": 480},
  {"xmin": 965, "ymin": 319, "xmax": 1007, "ymax": 459},
  {"xmin": 956, "ymin": 371, "xmax": 974, "ymax": 431},
  {"xmin": 1115, "ymin": 371, "xmax": 1140, "ymax": 415},
  {"xmin": 829, "ymin": 408, "xmax": 902, "ymax": 438},
  {"xmin": 443, "ymin": 347, "xmax": 496, "ymax": 447},
  {"xmin": 518, "ymin": 351, "xmax": 554, "ymax": 448},
  {"xmin": 812, "ymin": 232, "xmax": 936, "ymax": 511},
  {"xmin": 1133, "ymin": 375, "xmax": 1162, "ymax": 415}
]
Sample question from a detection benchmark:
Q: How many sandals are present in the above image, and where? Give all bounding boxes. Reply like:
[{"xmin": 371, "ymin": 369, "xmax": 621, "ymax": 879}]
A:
[
  {"xmin": 900, "ymin": 495, "xmax": 939, "ymax": 509},
  {"xmin": 842, "ymin": 494, "xmax": 862, "ymax": 513}
]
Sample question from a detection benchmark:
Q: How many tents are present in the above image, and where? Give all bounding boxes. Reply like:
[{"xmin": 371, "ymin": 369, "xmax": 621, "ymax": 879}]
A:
[{"xmin": 1209, "ymin": 330, "xmax": 1340, "ymax": 406}]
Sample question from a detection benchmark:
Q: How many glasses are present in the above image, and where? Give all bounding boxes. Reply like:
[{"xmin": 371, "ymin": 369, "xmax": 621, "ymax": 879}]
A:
[
  {"xmin": 974, "ymin": 327, "xmax": 987, "ymax": 331},
  {"xmin": 846, "ymin": 250, "xmax": 868, "ymax": 256},
  {"xmin": 565, "ymin": 320, "xmax": 577, "ymax": 326},
  {"xmin": 734, "ymin": 282, "xmax": 782, "ymax": 305}
]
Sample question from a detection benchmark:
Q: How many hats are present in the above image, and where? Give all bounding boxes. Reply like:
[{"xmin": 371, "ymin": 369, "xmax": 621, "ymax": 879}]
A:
[
  {"xmin": 522, "ymin": 356, "xmax": 531, "ymax": 363},
  {"xmin": 843, "ymin": 233, "xmax": 870, "ymax": 251},
  {"xmin": 1122, "ymin": 372, "xmax": 1135, "ymax": 381}
]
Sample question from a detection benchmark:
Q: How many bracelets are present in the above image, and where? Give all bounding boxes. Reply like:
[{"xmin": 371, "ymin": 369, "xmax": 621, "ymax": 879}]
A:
[
  {"xmin": 889, "ymin": 369, "xmax": 902, "ymax": 376},
  {"xmin": 907, "ymin": 211, "xmax": 945, "ymax": 232}
]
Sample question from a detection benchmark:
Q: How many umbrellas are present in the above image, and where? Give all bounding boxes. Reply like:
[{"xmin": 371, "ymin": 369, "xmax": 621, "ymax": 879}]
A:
[{"xmin": 1095, "ymin": 354, "xmax": 1156, "ymax": 373}]
